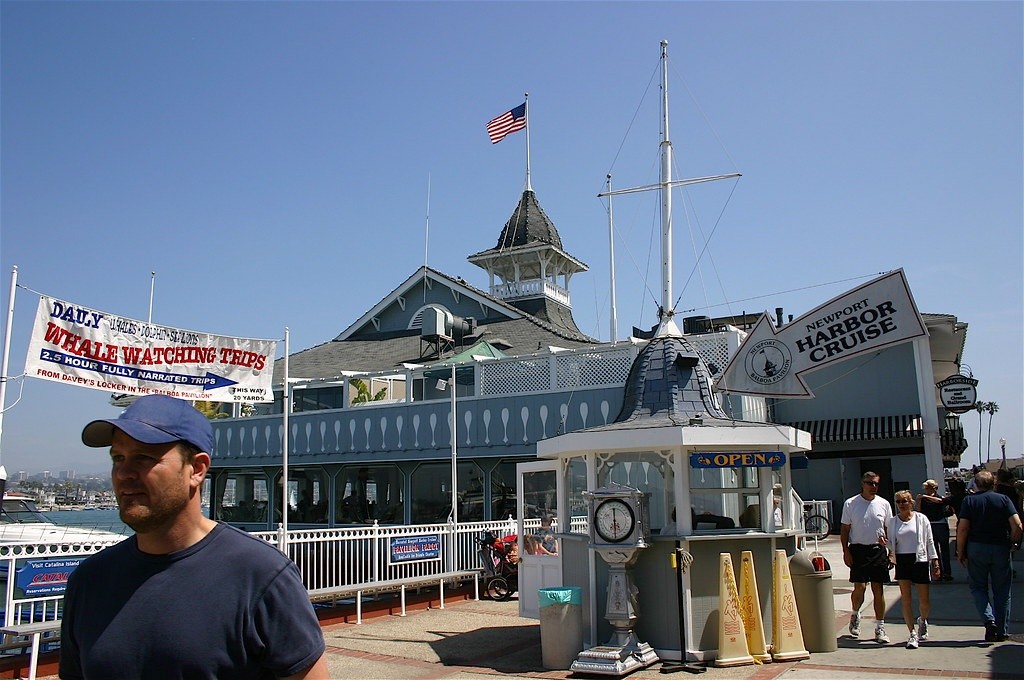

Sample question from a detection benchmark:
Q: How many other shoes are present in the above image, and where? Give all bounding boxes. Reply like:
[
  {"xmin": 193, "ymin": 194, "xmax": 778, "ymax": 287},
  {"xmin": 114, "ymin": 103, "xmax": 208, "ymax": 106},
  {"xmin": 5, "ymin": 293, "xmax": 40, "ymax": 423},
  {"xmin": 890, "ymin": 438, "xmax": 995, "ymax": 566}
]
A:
[
  {"xmin": 943, "ymin": 576, "xmax": 954, "ymax": 581},
  {"xmin": 997, "ymin": 633, "xmax": 1009, "ymax": 642},
  {"xmin": 935, "ymin": 577, "xmax": 943, "ymax": 581},
  {"xmin": 984, "ymin": 620, "xmax": 998, "ymax": 642}
]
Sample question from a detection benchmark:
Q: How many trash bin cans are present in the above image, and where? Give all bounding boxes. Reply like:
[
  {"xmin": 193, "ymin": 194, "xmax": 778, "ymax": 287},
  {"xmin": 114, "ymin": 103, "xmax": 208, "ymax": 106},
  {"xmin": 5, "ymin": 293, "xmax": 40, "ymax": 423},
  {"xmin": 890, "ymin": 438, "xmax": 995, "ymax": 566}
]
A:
[
  {"xmin": 538, "ymin": 587, "xmax": 584, "ymax": 670},
  {"xmin": 789, "ymin": 550, "xmax": 838, "ymax": 654}
]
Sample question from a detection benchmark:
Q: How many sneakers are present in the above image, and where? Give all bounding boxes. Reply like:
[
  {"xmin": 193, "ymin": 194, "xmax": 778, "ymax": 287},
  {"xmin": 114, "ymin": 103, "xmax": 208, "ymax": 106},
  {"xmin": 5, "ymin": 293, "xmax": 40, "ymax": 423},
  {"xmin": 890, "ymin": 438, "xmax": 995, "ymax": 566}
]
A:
[
  {"xmin": 875, "ymin": 628, "xmax": 890, "ymax": 643},
  {"xmin": 907, "ymin": 633, "xmax": 919, "ymax": 648},
  {"xmin": 849, "ymin": 613, "xmax": 861, "ymax": 636},
  {"xmin": 917, "ymin": 616, "xmax": 929, "ymax": 640}
]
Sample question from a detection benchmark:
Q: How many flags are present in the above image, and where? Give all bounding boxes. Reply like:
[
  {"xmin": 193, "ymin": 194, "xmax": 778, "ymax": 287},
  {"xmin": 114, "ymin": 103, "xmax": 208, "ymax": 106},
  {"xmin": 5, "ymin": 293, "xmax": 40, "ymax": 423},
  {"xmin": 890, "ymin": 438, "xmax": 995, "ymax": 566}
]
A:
[{"xmin": 487, "ymin": 101, "xmax": 527, "ymax": 144}]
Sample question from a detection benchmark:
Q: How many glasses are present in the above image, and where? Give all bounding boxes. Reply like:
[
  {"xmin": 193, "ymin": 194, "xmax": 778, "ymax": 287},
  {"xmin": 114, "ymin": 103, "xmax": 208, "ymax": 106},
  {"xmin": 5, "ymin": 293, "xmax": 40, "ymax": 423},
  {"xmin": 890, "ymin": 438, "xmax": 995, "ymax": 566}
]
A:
[
  {"xmin": 863, "ymin": 481, "xmax": 879, "ymax": 486},
  {"xmin": 896, "ymin": 499, "xmax": 910, "ymax": 503}
]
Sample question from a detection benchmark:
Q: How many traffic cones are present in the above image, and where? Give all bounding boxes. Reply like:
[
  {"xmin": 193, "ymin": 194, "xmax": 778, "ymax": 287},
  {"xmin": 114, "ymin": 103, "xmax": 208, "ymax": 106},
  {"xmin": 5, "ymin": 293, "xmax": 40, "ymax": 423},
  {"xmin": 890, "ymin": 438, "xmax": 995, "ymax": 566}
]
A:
[
  {"xmin": 737, "ymin": 550, "xmax": 773, "ymax": 664},
  {"xmin": 712, "ymin": 552, "xmax": 754, "ymax": 669},
  {"xmin": 768, "ymin": 549, "xmax": 810, "ymax": 662}
]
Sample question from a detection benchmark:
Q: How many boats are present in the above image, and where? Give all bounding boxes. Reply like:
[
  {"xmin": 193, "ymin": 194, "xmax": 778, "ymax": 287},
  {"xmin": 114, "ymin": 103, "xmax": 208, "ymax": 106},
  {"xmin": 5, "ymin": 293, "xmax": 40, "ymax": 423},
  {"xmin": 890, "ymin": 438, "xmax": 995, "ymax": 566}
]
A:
[{"xmin": 0, "ymin": 492, "xmax": 130, "ymax": 621}]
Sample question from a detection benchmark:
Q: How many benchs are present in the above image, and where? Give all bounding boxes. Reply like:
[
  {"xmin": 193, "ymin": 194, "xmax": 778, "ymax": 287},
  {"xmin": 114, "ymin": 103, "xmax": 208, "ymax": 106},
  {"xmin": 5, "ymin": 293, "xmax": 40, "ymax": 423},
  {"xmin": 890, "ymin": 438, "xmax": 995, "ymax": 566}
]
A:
[{"xmin": 0, "ymin": 568, "xmax": 487, "ymax": 680}]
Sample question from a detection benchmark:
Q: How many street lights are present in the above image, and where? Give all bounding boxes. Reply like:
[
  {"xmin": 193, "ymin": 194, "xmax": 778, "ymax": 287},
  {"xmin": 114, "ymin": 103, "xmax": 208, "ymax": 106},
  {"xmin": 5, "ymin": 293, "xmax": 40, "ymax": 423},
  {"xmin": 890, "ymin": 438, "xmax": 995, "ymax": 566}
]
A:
[{"xmin": 998, "ymin": 436, "xmax": 1009, "ymax": 472}]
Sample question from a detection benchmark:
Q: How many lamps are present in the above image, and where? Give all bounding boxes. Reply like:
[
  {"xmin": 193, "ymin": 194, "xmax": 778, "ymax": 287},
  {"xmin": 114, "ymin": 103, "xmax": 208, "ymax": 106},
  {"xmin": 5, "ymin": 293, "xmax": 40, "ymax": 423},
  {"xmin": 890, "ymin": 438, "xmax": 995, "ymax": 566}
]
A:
[
  {"xmin": 960, "ymin": 363, "xmax": 973, "ymax": 379},
  {"xmin": 939, "ymin": 412, "xmax": 959, "ymax": 445}
]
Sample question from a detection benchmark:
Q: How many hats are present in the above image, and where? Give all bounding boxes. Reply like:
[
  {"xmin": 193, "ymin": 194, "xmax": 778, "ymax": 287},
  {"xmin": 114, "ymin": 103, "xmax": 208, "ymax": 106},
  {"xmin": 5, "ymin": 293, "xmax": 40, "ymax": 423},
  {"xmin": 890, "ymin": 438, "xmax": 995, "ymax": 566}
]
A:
[{"xmin": 81, "ymin": 394, "xmax": 214, "ymax": 455}]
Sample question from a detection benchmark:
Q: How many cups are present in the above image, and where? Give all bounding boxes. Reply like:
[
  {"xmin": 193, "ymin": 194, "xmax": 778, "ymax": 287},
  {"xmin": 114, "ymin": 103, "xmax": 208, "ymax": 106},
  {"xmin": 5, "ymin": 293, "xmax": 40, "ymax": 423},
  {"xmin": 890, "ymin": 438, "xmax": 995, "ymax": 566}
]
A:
[{"xmin": 813, "ymin": 556, "xmax": 824, "ymax": 572}]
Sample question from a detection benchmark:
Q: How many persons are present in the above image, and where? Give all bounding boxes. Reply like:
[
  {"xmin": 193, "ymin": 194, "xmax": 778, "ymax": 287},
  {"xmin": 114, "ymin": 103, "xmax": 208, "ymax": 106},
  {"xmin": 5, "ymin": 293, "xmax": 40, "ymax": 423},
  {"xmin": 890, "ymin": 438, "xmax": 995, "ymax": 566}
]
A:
[
  {"xmin": 967, "ymin": 467, "xmax": 981, "ymax": 494},
  {"xmin": 57, "ymin": 395, "xmax": 327, "ymax": 680},
  {"xmin": 996, "ymin": 470, "xmax": 1019, "ymax": 509},
  {"xmin": 343, "ymin": 490, "xmax": 358, "ymax": 504},
  {"xmin": 887, "ymin": 490, "xmax": 940, "ymax": 648},
  {"xmin": 510, "ymin": 542, "xmax": 518, "ymax": 560},
  {"xmin": 660, "ymin": 506, "xmax": 677, "ymax": 535},
  {"xmin": 742, "ymin": 488, "xmax": 782, "ymax": 528},
  {"xmin": 841, "ymin": 472, "xmax": 896, "ymax": 644},
  {"xmin": 535, "ymin": 518, "xmax": 558, "ymax": 556},
  {"xmin": 298, "ymin": 490, "xmax": 311, "ymax": 512},
  {"xmin": 956, "ymin": 470, "xmax": 1022, "ymax": 640},
  {"xmin": 916, "ymin": 479, "xmax": 954, "ymax": 581}
]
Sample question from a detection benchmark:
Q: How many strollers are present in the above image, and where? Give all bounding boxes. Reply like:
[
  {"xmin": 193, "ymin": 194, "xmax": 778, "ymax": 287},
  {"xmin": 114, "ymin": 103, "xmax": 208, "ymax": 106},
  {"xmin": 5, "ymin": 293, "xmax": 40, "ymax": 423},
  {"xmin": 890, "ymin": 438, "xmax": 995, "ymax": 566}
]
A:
[{"xmin": 472, "ymin": 528, "xmax": 546, "ymax": 602}]
